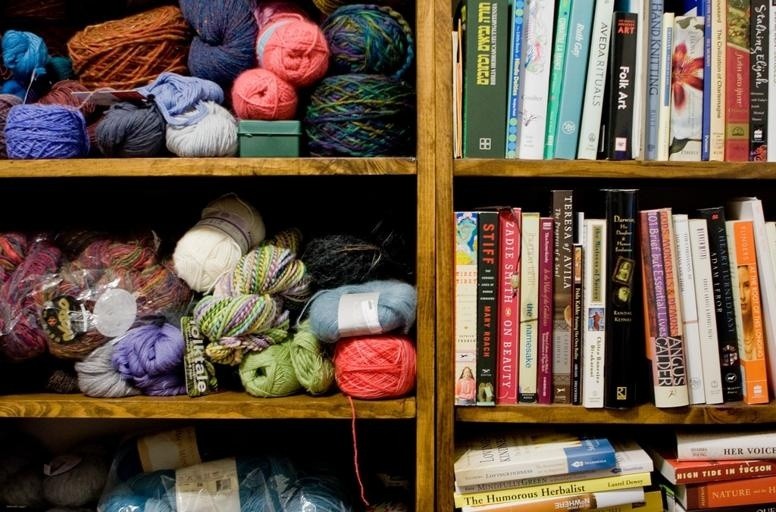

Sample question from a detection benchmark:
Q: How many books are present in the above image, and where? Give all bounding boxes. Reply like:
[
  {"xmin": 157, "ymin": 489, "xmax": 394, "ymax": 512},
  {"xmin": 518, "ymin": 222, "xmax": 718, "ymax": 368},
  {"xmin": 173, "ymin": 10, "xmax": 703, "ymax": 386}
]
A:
[
  {"xmin": 452, "ymin": 183, "xmax": 775, "ymax": 414},
  {"xmin": 451, "ymin": 427, "xmax": 775, "ymax": 511},
  {"xmin": 451, "ymin": 1, "xmax": 776, "ymax": 162}
]
